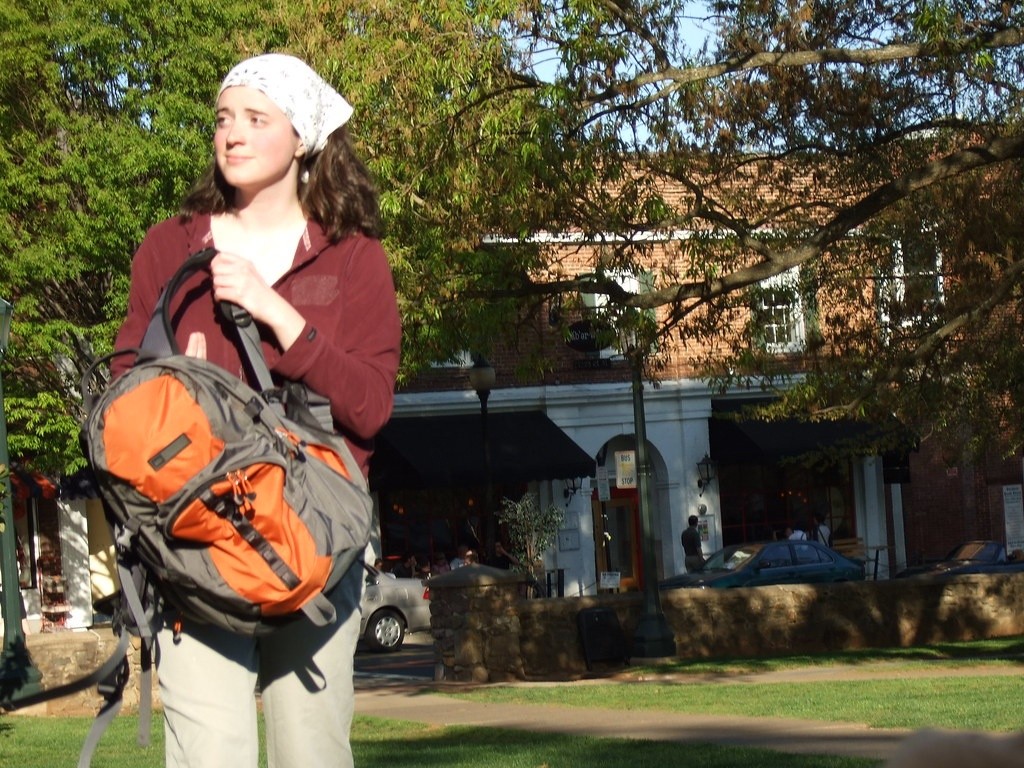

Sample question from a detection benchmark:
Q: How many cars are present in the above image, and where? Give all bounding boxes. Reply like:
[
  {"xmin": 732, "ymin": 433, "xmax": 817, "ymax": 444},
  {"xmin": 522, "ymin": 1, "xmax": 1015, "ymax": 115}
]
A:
[
  {"xmin": 356, "ymin": 561, "xmax": 433, "ymax": 652},
  {"xmin": 894, "ymin": 537, "xmax": 1024, "ymax": 575},
  {"xmin": 656, "ymin": 538, "xmax": 867, "ymax": 591}
]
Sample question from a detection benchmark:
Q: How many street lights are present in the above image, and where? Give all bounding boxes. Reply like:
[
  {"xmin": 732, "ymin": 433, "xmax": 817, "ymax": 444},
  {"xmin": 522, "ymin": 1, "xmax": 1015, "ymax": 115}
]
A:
[
  {"xmin": 615, "ymin": 305, "xmax": 680, "ymax": 659},
  {"xmin": 467, "ymin": 351, "xmax": 500, "ymax": 564}
]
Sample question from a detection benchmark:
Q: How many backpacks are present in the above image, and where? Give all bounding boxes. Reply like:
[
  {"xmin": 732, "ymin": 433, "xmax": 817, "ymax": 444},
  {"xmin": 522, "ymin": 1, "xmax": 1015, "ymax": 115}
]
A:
[{"xmin": 75, "ymin": 248, "xmax": 380, "ymax": 634}]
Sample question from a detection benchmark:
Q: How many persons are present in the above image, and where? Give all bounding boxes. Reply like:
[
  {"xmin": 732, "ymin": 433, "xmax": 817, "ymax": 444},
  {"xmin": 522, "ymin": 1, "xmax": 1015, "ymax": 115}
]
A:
[
  {"xmin": 785, "ymin": 527, "xmax": 807, "ymax": 541},
  {"xmin": 107, "ymin": 54, "xmax": 402, "ymax": 768},
  {"xmin": 373, "ymin": 550, "xmax": 477, "ymax": 583},
  {"xmin": 813, "ymin": 511, "xmax": 831, "ymax": 546},
  {"xmin": 681, "ymin": 515, "xmax": 705, "ymax": 573},
  {"xmin": 35, "ymin": 542, "xmax": 65, "ymax": 623}
]
